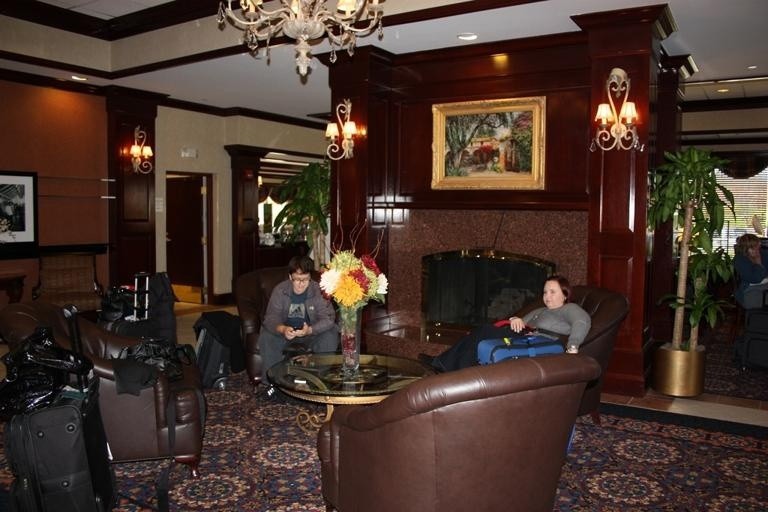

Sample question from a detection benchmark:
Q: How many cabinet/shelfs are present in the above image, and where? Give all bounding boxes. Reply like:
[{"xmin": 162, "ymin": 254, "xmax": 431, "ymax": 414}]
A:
[{"xmin": 258, "ymin": 245, "xmax": 293, "ymax": 269}]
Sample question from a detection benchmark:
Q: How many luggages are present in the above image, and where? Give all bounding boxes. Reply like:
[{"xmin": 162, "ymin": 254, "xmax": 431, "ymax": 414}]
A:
[
  {"xmin": 477, "ymin": 325, "xmax": 574, "ymax": 453},
  {"xmin": 10, "ymin": 305, "xmax": 115, "ymax": 511},
  {"xmin": 112, "ymin": 273, "xmax": 160, "ymax": 339},
  {"xmin": 194, "ymin": 329, "xmax": 232, "ymax": 389}
]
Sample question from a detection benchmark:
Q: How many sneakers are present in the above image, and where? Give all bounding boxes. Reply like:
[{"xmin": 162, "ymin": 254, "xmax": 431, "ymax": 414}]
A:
[
  {"xmin": 418, "ymin": 353, "xmax": 442, "ymax": 374},
  {"xmin": 265, "ymin": 384, "xmax": 277, "ymax": 402}
]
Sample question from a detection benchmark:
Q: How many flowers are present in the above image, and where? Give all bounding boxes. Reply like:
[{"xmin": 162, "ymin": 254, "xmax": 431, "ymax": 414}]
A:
[{"xmin": 319, "ymin": 209, "xmax": 389, "ymax": 328}]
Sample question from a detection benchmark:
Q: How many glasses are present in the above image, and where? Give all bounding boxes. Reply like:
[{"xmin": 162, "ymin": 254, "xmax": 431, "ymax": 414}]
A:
[{"xmin": 291, "ymin": 274, "xmax": 310, "ymax": 282}]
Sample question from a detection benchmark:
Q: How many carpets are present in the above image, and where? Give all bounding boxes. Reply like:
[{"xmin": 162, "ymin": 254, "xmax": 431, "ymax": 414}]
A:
[{"xmin": 0, "ymin": 367, "xmax": 768, "ymax": 512}]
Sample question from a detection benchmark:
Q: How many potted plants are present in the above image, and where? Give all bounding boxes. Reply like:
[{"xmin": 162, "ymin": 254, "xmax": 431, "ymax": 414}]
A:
[{"xmin": 646, "ymin": 145, "xmax": 736, "ymax": 398}]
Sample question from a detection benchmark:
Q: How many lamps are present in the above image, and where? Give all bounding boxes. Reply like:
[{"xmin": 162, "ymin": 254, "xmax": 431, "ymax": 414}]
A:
[
  {"xmin": 129, "ymin": 124, "xmax": 154, "ymax": 174},
  {"xmin": 325, "ymin": 98, "xmax": 359, "ymax": 161},
  {"xmin": 752, "ymin": 214, "xmax": 765, "ymax": 236},
  {"xmin": 215, "ymin": 0, "xmax": 386, "ymax": 76},
  {"xmin": 589, "ymin": 67, "xmax": 647, "ymax": 152}
]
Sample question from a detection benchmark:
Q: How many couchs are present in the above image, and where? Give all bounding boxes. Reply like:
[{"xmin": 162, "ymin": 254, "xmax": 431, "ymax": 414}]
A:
[
  {"xmin": 0, "ymin": 301, "xmax": 207, "ymax": 478},
  {"xmin": 506, "ymin": 285, "xmax": 631, "ymax": 426},
  {"xmin": 317, "ymin": 354, "xmax": 601, "ymax": 511},
  {"xmin": 235, "ymin": 266, "xmax": 322, "ymax": 394}
]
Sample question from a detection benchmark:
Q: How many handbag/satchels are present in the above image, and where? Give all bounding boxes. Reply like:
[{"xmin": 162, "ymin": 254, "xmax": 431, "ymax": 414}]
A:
[
  {"xmin": 117, "ymin": 337, "xmax": 192, "ymax": 382},
  {"xmin": 101, "ymin": 284, "xmax": 151, "ymax": 321},
  {"xmin": 0, "ymin": 327, "xmax": 94, "ymax": 421}
]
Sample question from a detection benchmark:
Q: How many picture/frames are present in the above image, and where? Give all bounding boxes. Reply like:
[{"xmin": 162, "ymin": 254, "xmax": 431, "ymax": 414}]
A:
[
  {"xmin": 0, "ymin": 170, "xmax": 39, "ymax": 251},
  {"xmin": 431, "ymin": 95, "xmax": 546, "ymax": 191}
]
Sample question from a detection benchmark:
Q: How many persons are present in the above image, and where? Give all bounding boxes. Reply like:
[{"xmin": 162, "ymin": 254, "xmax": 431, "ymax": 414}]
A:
[
  {"xmin": 733, "ymin": 234, "xmax": 767, "ymax": 302},
  {"xmin": 258, "ymin": 255, "xmax": 339, "ymax": 386},
  {"xmin": 418, "ymin": 275, "xmax": 591, "ymax": 373}
]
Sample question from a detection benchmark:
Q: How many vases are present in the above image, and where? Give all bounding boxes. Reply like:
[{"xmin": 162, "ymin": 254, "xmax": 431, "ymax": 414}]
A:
[{"xmin": 338, "ymin": 307, "xmax": 362, "ymax": 377}]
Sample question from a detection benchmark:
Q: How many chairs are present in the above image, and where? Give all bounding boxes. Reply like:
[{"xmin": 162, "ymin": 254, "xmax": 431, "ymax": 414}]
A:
[{"xmin": 32, "ymin": 253, "xmax": 104, "ymax": 323}]
